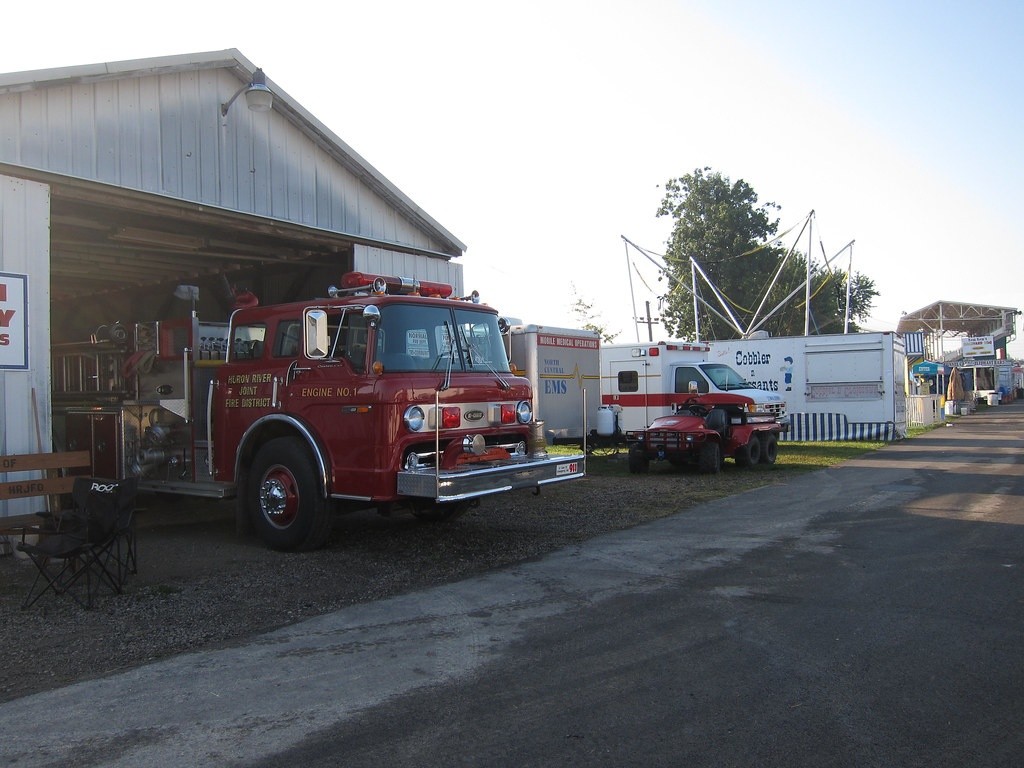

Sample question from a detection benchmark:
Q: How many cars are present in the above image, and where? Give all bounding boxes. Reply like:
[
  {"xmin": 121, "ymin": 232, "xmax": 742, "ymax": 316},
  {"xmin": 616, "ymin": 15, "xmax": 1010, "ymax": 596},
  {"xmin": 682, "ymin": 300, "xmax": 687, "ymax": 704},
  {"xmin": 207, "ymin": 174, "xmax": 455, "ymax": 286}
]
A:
[{"xmin": 626, "ymin": 393, "xmax": 782, "ymax": 475}]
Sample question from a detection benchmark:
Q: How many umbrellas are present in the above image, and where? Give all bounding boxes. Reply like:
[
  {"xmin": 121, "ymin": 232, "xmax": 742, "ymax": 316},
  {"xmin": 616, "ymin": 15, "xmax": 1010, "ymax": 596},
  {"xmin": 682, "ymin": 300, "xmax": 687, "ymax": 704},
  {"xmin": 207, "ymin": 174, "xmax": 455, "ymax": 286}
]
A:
[{"xmin": 948, "ymin": 367, "xmax": 964, "ymax": 415}]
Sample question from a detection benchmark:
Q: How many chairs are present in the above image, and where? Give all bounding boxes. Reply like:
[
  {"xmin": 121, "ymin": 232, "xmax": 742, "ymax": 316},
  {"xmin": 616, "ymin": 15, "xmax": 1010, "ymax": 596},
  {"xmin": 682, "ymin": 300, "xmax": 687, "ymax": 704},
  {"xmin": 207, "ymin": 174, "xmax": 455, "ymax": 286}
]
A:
[
  {"xmin": 704, "ymin": 408, "xmax": 728, "ymax": 439},
  {"xmin": 17, "ymin": 474, "xmax": 143, "ymax": 611},
  {"xmin": 675, "ymin": 410, "xmax": 694, "ymax": 416},
  {"xmin": 380, "ymin": 353, "xmax": 417, "ymax": 373}
]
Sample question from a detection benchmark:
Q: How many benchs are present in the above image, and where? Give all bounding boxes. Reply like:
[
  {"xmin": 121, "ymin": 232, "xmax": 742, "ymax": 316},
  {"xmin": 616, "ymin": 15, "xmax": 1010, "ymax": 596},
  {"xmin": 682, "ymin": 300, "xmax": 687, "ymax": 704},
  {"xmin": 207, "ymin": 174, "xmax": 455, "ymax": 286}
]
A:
[{"xmin": 0, "ymin": 450, "xmax": 92, "ymax": 535}]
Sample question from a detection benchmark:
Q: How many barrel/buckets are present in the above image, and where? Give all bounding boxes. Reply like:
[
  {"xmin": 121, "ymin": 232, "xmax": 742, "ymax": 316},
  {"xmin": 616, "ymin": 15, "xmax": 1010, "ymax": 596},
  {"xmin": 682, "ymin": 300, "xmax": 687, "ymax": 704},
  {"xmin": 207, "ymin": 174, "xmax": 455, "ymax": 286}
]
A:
[
  {"xmin": 597, "ymin": 407, "xmax": 613, "ymax": 436},
  {"xmin": 987, "ymin": 393, "xmax": 998, "ymax": 406}
]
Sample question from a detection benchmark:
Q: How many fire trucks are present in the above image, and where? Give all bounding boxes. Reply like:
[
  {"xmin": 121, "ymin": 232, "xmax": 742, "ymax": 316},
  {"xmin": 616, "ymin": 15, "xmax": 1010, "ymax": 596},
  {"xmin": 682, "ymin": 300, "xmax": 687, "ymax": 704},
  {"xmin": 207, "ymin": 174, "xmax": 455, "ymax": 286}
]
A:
[{"xmin": 51, "ymin": 272, "xmax": 586, "ymax": 550}]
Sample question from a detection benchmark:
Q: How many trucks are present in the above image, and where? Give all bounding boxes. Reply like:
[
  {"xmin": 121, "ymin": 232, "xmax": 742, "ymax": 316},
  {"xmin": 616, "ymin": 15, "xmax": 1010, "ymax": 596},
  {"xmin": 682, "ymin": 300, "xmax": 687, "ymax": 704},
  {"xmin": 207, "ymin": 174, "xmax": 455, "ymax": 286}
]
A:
[{"xmin": 548, "ymin": 341, "xmax": 790, "ymax": 445}]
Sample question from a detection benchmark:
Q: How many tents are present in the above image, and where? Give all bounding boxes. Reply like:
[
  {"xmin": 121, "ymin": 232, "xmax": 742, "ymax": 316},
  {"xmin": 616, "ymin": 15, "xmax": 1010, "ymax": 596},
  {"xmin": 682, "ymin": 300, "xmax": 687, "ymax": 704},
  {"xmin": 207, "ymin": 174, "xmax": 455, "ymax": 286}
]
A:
[{"xmin": 913, "ymin": 361, "xmax": 952, "ymax": 394}]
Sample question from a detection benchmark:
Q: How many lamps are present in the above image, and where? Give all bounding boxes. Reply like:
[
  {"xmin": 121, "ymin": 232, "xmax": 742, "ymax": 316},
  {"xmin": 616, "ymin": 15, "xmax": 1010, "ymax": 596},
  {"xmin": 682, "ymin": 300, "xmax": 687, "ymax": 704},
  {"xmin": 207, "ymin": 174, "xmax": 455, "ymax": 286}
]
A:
[{"xmin": 220, "ymin": 66, "xmax": 275, "ymax": 117}]
